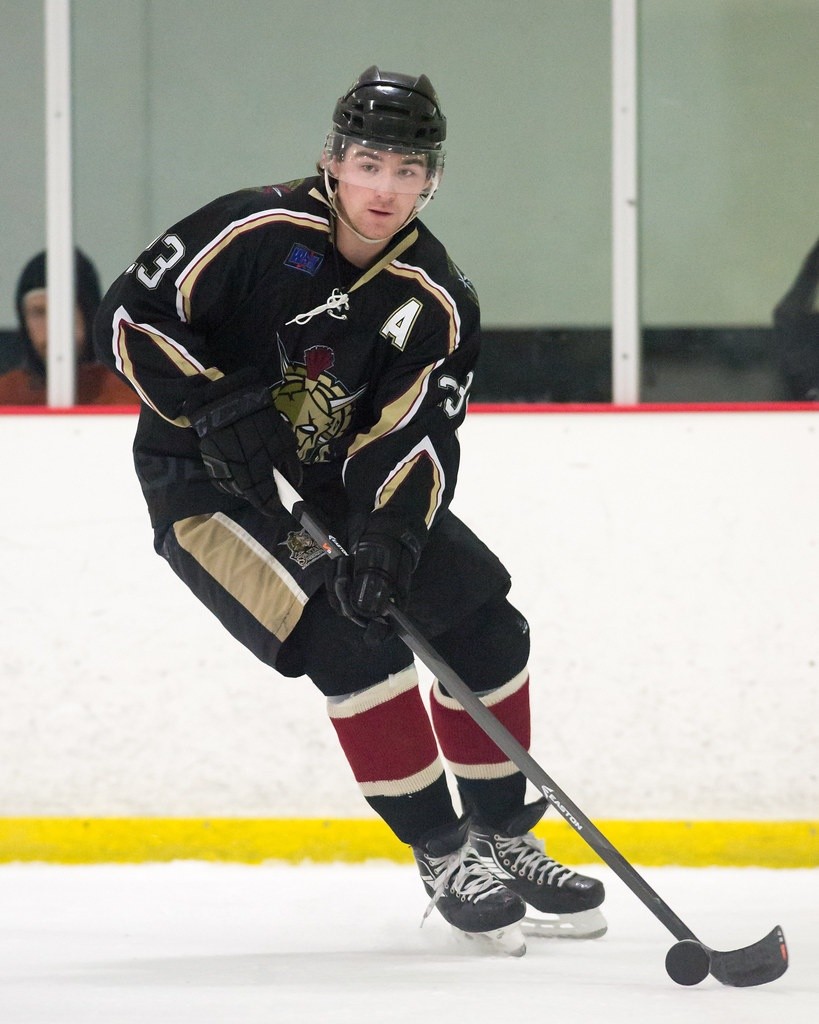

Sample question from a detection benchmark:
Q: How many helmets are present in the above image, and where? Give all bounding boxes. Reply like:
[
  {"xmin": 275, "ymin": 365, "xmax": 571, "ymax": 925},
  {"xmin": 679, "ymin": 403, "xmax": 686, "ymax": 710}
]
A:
[{"xmin": 321, "ymin": 65, "xmax": 446, "ymax": 195}]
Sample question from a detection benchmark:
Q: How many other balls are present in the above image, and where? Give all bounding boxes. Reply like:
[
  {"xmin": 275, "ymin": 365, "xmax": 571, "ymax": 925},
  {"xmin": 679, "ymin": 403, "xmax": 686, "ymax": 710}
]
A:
[{"xmin": 665, "ymin": 939, "xmax": 711, "ymax": 986}]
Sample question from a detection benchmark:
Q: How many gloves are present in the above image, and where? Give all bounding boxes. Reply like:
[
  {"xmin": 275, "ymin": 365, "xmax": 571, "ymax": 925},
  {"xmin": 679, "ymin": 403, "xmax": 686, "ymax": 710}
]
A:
[
  {"xmin": 333, "ymin": 503, "xmax": 428, "ymax": 647},
  {"xmin": 182, "ymin": 366, "xmax": 303, "ymax": 518}
]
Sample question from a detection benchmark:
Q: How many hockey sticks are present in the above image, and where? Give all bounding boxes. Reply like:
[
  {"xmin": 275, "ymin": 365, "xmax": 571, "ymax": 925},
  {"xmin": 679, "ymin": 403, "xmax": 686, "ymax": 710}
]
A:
[{"xmin": 273, "ymin": 462, "xmax": 788, "ymax": 988}]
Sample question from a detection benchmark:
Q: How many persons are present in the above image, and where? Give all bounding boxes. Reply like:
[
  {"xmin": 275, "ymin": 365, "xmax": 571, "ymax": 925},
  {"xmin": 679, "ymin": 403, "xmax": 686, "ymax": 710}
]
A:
[
  {"xmin": 0, "ymin": 246, "xmax": 142, "ymax": 405},
  {"xmin": 92, "ymin": 64, "xmax": 606, "ymax": 933}
]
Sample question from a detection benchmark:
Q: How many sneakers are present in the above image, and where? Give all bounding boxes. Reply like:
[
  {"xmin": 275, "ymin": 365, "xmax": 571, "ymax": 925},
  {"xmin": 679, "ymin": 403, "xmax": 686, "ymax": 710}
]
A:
[
  {"xmin": 410, "ymin": 802, "xmax": 527, "ymax": 957},
  {"xmin": 468, "ymin": 795, "xmax": 608, "ymax": 940}
]
